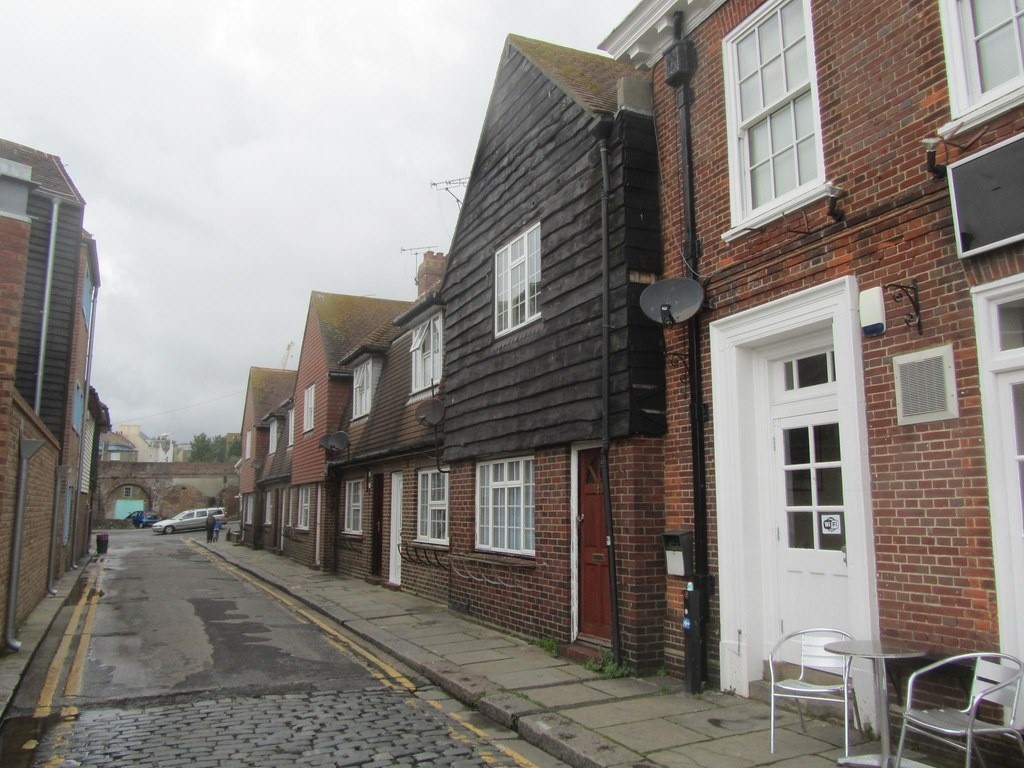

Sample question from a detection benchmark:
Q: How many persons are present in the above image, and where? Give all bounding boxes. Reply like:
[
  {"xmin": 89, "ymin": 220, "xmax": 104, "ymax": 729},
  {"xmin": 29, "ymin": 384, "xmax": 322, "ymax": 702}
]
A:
[{"xmin": 206, "ymin": 512, "xmax": 220, "ymax": 543}]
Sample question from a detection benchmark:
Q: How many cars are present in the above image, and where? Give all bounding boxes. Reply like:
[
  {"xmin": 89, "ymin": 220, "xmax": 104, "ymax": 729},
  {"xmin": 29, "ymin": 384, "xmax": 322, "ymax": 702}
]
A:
[{"xmin": 125, "ymin": 510, "xmax": 161, "ymax": 528}]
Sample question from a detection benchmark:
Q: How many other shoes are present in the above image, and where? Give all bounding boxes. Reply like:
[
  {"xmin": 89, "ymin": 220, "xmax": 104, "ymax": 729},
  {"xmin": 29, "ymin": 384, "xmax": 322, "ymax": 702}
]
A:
[
  {"xmin": 213, "ymin": 539, "xmax": 215, "ymax": 542},
  {"xmin": 210, "ymin": 540, "xmax": 212, "ymax": 543},
  {"xmin": 216, "ymin": 538, "xmax": 218, "ymax": 542},
  {"xmin": 207, "ymin": 540, "xmax": 209, "ymax": 543}
]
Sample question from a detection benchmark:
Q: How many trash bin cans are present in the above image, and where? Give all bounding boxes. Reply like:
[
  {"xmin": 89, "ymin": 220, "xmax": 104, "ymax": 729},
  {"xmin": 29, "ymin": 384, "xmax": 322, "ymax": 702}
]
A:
[{"xmin": 96, "ymin": 533, "xmax": 109, "ymax": 554}]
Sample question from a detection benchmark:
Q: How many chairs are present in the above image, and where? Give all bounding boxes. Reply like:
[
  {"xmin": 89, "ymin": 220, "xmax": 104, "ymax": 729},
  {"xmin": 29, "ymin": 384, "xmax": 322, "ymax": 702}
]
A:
[
  {"xmin": 767, "ymin": 628, "xmax": 862, "ymax": 761},
  {"xmin": 891, "ymin": 652, "xmax": 1024, "ymax": 768}
]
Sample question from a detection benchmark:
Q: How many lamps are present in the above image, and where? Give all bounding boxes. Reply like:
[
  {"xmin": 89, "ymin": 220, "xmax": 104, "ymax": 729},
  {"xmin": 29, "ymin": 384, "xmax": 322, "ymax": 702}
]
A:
[
  {"xmin": 920, "ymin": 137, "xmax": 946, "ymax": 179},
  {"xmin": 825, "ymin": 183, "xmax": 845, "ymax": 221}
]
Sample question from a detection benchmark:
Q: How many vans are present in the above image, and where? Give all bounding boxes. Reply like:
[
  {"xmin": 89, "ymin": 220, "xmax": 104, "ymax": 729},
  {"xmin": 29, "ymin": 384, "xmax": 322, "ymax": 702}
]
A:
[{"xmin": 152, "ymin": 507, "xmax": 228, "ymax": 535}]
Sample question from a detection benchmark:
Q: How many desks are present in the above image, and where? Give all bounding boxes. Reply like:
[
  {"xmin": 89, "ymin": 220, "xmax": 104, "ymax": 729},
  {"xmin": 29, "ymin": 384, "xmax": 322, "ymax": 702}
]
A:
[{"xmin": 823, "ymin": 637, "xmax": 931, "ymax": 768}]
